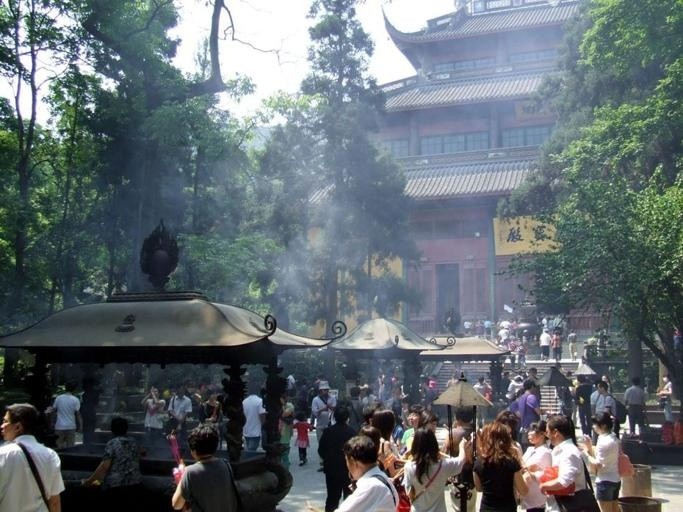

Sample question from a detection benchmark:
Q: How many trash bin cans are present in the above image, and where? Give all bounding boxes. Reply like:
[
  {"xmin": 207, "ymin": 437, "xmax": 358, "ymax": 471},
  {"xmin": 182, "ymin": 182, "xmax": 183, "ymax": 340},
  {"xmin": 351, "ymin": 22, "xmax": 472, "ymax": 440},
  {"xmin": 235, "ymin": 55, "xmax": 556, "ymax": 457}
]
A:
[
  {"xmin": 621, "ymin": 463, "xmax": 651, "ymax": 497},
  {"xmin": 617, "ymin": 497, "xmax": 661, "ymax": 512}
]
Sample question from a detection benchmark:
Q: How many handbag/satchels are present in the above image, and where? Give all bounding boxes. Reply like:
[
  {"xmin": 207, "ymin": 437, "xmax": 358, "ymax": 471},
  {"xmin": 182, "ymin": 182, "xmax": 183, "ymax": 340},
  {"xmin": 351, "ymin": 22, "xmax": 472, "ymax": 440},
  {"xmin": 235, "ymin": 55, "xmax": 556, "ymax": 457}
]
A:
[
  {"xmin": 519, "ymin": 427, "xmax": 529, "ymax": 444},
  {"xmin": 281, "ymin": 415, "xmax": 295, "ymax": 425},
  {"xmin": 556, "ymin": 489, "xmax": 601, "ymax": 512},
  {"xmin": 396, "ymin": 484, "xmax": 410, "ymax": 512},
  {"xmin": 604, "ymin": 395, "xmax": 626, "ymax": 424},
  {"xmin": 618, "ymin": 454, "xmax": 634, "ymax": 478}
]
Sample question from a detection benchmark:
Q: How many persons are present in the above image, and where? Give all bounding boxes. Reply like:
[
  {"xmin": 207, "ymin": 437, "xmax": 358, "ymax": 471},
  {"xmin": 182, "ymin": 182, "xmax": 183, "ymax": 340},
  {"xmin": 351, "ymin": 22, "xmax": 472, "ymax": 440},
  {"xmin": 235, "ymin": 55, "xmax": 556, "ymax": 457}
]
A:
[
  {"xmin": 172, "ymin": 429, "xmax": 239, "ymax": 511},
  {"xmin": 554, "ymin": 362, "xmax": 674, "ymax": 444},
  {"xmin": 32, "ymin": 379, "xmax": 100, "ymax": 447},
  {"xmin": 242, "ymin": 386, "xmax": 267, "ymax": 450},
  {"xmin": 472, "ymin": 368, "xmax": 550, "ymax": 432},
  {"xmin": 84, "ymin": 416, "xmax": 144, "ymax": 511},
  {"xmin": 348, "ymin": 371, "xmax": 455, "ymax": 433},
  {"xmin": 0, "ymin": 403, "xmax": 64, "ymax": 511},
  {"xmin": 279, "ymin": 373, "xmax": 337, "ymax": 472},
  {"xmin": 318, "ymin": 407, "xmax": 622, "ymax": 512},
  {"xmin": 464, "ymin": 320, "xmax": 603, "ymax": 363},
  {"xmin": 142, "ymin": 386, "xmax": 221, "ymax": 434}
]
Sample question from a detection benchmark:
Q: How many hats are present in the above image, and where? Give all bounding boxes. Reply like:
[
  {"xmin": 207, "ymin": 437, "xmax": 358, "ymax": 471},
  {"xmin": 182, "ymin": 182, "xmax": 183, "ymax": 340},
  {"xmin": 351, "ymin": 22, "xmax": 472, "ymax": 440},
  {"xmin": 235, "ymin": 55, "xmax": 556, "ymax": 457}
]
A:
[{"xmin": 318, "ymin": 381, "xmax": 330, "ymax": 390}]
